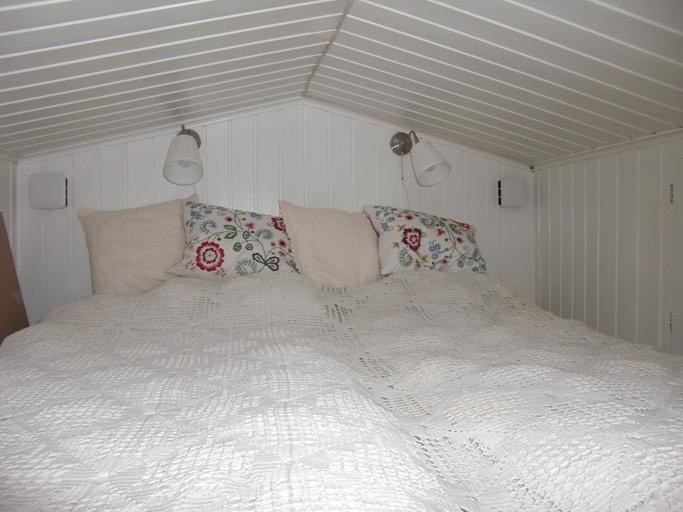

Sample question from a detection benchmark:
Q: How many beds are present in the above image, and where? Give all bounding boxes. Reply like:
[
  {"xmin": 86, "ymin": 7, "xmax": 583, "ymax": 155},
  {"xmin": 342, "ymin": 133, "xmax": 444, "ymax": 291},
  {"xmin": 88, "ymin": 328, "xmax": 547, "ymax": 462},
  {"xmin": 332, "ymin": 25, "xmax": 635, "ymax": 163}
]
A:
[{"xmin": 0, "ymin": 295, "xmax": 682, "ymax": 511}]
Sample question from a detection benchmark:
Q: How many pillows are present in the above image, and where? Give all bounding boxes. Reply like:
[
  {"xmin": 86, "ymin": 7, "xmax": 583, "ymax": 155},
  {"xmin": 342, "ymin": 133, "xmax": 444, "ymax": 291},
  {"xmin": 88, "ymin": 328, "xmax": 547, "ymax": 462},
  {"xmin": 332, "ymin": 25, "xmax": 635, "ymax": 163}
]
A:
[
  {"xmin": 77, "ymin": 193, "xmax": 198, "ymax": 293},
  {"xmin": 278, "ymin": 200, "xmax": 379, "ymax": 283},
  {"xmin": 364, "ymin": 204, "xmax": 486, "ymax": 275},
  {"xmin": 166, "ymin": 200, "xmax": 300, "ymax": 281}
]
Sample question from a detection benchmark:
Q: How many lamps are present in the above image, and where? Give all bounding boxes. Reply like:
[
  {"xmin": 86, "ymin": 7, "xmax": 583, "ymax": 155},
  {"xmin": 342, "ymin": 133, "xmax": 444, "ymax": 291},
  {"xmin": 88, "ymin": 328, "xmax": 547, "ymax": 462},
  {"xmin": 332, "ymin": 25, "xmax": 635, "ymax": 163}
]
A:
[
  {"xmin": 162, "ymin": 126, "xmax": 203, "ymax": 185},
  {"xmin": 390, "ymin": 130, "xmax": 450, "ymax": 186}
]
